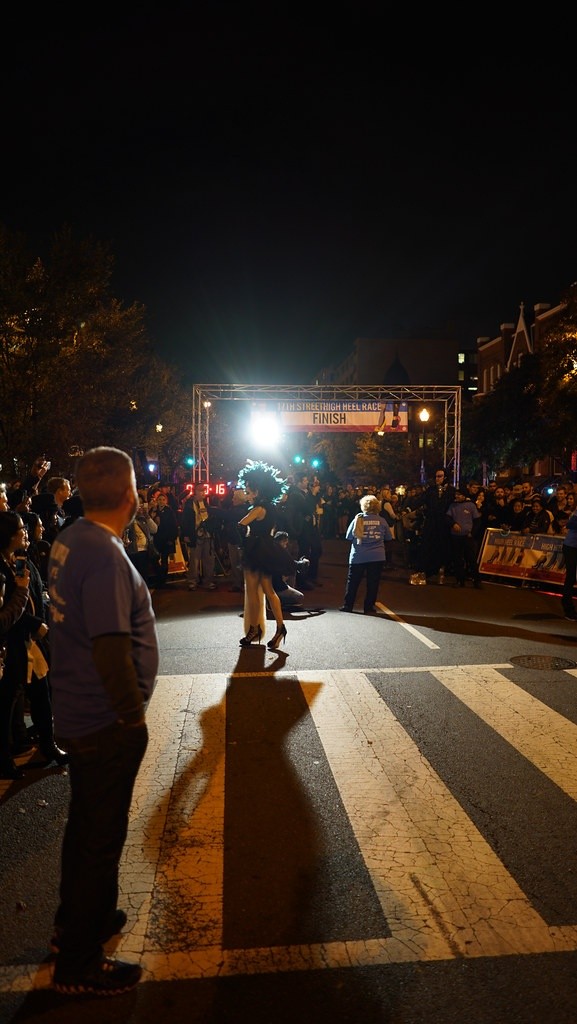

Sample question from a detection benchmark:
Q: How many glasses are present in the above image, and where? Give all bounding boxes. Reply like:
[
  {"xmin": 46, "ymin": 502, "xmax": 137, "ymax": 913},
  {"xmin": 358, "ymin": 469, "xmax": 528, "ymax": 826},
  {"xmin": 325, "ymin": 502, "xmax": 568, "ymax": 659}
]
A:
[{"xmin": 19, "ymin": 523, "xmax": 28, "ymax": 531}]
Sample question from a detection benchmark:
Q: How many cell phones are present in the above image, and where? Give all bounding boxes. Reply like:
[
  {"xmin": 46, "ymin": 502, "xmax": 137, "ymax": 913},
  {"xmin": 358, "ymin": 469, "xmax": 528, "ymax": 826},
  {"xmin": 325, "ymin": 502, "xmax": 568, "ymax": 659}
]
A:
[
  {"xmin": 16, "ymin": 556, "xmax": 26, "ymax": 578},
  {"xmin": 45, "ymin": 462, "xmax": 51, "ymax": 470}
]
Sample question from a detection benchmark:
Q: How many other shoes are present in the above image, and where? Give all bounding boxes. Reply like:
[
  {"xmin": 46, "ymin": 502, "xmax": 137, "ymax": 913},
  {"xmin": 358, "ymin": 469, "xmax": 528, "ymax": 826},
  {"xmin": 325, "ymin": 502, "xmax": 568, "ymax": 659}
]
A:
[
  {"xmin": 307, "ymin": 577, "xmax": 323, "ymax": 587},
  {"xmin": 407, "ymin": 572, "xmax": 428, "ymax": 585},
  {"xmin": 459, "ymin": 579, "xmax": 468, "ymax": 588},
  {"xmin": 186, "ymin": 580, "xmax": 197, "ymax": 591},
  {"xmin": 0, "ymin": 758, "xmax": 26, "ymax": 779},
  {"xmin": 206, "ymin": 582, "xmax": 217, "ymax": 589},
  {"xmin": 300, "ymin": 579, "xmax": 315, "ymax": 591},
  {"xmin": 39, "ymin": 740, "xmax": 70, "ymax": 764},
  {"xmin": 437, "ymin": 572, "xmax": 448, "ymax": 585},
  {"xmin": 10, "ymin": 740, "xmax": 33, "ymax": 755},
  {"xmin": 364, "ymin": 609, "xmax": 376, "ymax": 615},
  {"xmin": 473, "ymin": 581, "xmax": 484, "ymax": 590},
  {"xmin": 339, "ymin": 606, "xmax": 351, "ymax": 612},
  {"xmin": 561, "ymin": 596, "xmax": 577, "ymax": 622}
]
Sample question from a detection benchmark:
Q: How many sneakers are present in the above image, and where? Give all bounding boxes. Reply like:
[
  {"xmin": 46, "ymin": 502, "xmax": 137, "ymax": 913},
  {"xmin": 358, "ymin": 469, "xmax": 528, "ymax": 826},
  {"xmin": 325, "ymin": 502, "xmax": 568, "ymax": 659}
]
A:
[
  {"xmin": 53, "ymin": 948, "xmax": 143, "ymax": 994},
  {"xmin": 49, "ymin": 910, "xmax": 127, "ymax": 951}
]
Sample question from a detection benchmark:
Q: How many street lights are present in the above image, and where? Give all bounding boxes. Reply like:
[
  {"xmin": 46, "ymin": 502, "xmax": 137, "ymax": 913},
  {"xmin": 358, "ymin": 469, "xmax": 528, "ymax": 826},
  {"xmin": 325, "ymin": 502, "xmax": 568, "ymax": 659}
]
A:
[
  {"xmin": 156, "ymin": 424, "xmax": 163, "ymax": 480},
  {"xmin": 419, "ymin": 408, "xmax": 430, "ymax": 482}
]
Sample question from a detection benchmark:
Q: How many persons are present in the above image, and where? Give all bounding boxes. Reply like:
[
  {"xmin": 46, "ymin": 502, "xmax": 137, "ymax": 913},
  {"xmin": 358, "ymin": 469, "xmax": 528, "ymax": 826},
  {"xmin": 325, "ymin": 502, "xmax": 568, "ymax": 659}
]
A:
[
  {"xmin": 323, "ymin": 480, "xmax": 423, "ymax": 570},
  {"xmin": 48, "ymin": 449, "xmax": 159, "ymax": 993},
  {"xmin": 401, "ymin": 466, "xmax": 577, "ymax": 621},
  {"xmin": 338, "ymin": 495, "xmax": 392, "ymax": 615},
  {"xmin": 0, "ymin": 456, "xmax": 84, "ymax": 779},
  {"xmin": 121, "ymin": 482, "xmax": 250, "ymax": 595},
  {"xmin": 238, "ymin": 459, "xmax": 323, "ymax": 648}
]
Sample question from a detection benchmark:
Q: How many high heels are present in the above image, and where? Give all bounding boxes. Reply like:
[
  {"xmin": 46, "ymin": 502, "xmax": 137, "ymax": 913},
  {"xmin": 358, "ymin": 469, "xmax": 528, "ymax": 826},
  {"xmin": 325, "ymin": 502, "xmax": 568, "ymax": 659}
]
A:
[
  {"xmin": 240, "ymin": 624, "xmax": 261, "ymax": 645},
  {"xmin": 268, "ymin": 625, "xmax": 286, "ymax": 649}
]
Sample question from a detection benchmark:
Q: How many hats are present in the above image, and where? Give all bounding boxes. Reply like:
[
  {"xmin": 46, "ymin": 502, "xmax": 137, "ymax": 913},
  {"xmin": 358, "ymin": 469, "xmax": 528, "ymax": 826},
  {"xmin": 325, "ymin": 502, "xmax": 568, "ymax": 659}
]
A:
[{"xmin": 274, "ymin": 531, "xmax": 287, "ymax": 539}]
